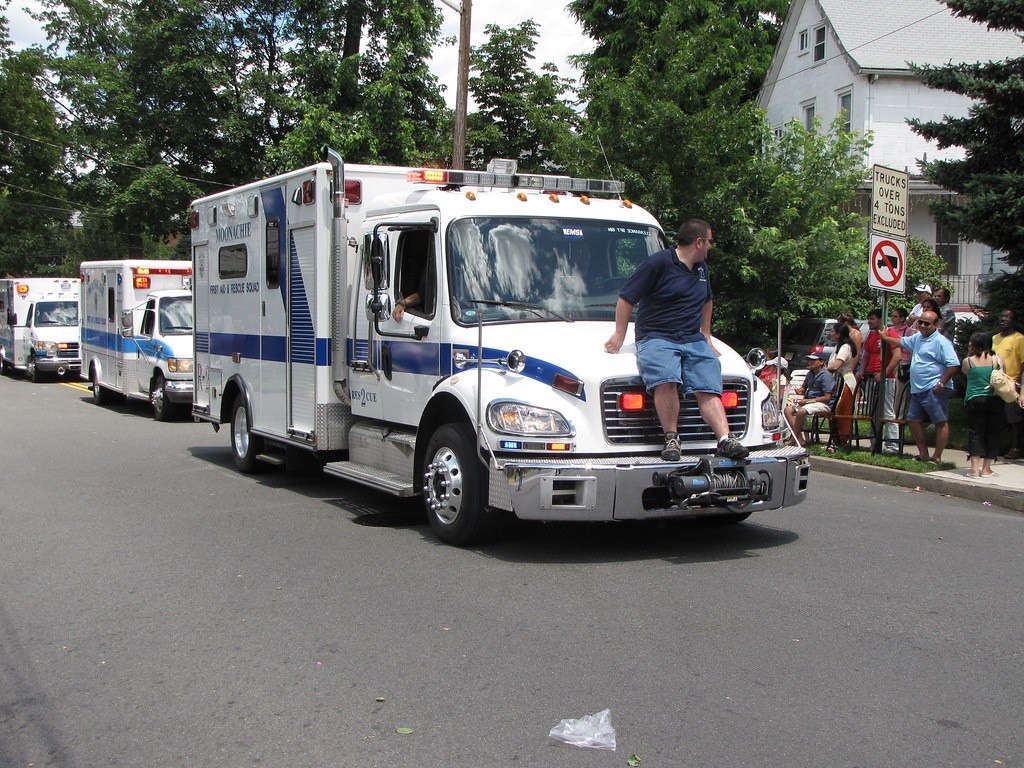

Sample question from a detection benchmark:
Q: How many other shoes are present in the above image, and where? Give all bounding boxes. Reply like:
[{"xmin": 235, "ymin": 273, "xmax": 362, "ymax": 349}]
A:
[
  {"xmin": 884, "ymin": 446, "xmax": 900, "ymax": 454},
  {"xmin": 828, "ymin": 446, "xmax": 849, "ymax": 454},
  {"xmin": 1004, "ymin": 449, "xmax": 1022, "ymax": 459}
]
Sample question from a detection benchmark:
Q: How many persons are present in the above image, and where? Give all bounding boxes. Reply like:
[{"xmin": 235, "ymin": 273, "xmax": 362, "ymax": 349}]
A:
[
  {"xmin": 604, "ymin": 217, "xmax": 752, "ymax": 462},
  {"xmin": 961, "ymin": 307, "xmax": 1024, "ymax": 480},
  {"xmin": 877, "ymin": 311, "xmax": 960, "ymax": 468},
  {"xmin": 758, "ymin": 284, "xmax": 956, "ymax": 448},
  {"xmin": 392, "ymin": 250, "xmax": 483, "ymax": 323}
]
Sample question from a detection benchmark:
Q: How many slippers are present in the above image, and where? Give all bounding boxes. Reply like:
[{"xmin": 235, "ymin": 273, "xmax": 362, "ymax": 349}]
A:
[
  {"xmin": 964, "ymin": 471, "xmax": 980, "ymax": 479},
  {"xmin": 927, "ymin": 458, "xmax": 941, "ymax": 465},
  {"xmin": 911, "ymin": 455, "xmax": 925, "ymax": 463},
  {"xmin": 978, "ymin": 469, "xmax": 999, "ymax": 478}
]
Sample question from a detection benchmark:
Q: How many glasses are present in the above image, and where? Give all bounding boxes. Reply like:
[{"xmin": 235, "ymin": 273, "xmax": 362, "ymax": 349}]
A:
[
  {"xmin": 892, "ymin": 314, "xmax": 902, "ymax": 318},
  {"xmin": 917, "ymin": 319, "xmax": 934, "ymax": 327},
  {"xmin": 702, "ymin": 238, "xmax": 714, "ymax": 244}
]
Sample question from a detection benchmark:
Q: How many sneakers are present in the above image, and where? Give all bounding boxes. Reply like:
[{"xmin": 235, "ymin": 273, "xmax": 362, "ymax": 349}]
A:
[
  {"xmin": 661, "ymin": 431, "xmax": 682, "ymax": 460},
  {"xmin": 716, "ymin": 437, "xmax": 749, "ymax": 460}
]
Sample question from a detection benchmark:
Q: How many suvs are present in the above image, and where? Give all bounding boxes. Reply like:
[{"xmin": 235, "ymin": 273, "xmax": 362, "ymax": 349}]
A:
[{"xmin": 780, "ymin": 316, "xmax": 873, "ymax": 372}]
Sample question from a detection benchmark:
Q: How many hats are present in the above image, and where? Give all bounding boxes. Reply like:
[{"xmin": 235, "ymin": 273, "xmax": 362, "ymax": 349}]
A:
[
  {"xmin": 804, "ymin": 352, "xmax": 825, "ymax": 361},
  {"xmin": 913, "ymin": 284, "xmax": 932, "ymax": 294},
  {"xmin": 766, "ymin": 358, "xmax": 788, "ymax": 369}
]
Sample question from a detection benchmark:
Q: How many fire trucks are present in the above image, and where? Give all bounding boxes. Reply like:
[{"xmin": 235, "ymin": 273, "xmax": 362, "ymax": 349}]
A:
[
  {"xmin": 78, "ymin": 259, "xmax": 195, "ymax": 422},
  {"xmin": 185, "ymin": 145, "xmax": 812, "ymax": 545},
  {"xmin": 0, "ymin": 277, "xmax": 81, "ymax": 383}
]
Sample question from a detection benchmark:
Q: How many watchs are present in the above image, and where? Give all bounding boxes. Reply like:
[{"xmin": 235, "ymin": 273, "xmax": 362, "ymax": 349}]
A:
[{"xmin": 938, "ymin": 381, "xmax": 944, "ymax": 387}]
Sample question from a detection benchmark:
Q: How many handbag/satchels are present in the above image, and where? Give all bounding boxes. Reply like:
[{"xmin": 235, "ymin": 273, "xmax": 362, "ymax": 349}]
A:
[{"xmin": 990, "ymin": 355, "xmax": 1018, "ymax": 402}]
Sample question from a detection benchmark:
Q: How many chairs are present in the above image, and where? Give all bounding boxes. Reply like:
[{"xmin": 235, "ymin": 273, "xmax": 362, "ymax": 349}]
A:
[
  {"xmin": 870, "ymin": 380, "xmax": 930, "ymax": 459},
  {"xmin": 792, "ymin": 369, "xmax": 844, "ymax": 445},
  {"xmin": 826, "ymin": 373, "xmax": 880, "ymax": 454}
]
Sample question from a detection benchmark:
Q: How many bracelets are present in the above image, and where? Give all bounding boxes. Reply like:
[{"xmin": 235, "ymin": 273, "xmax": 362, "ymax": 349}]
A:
[{"xmin": 395, "ymin": 301, "xmax": 405, "ymax": 306}]
